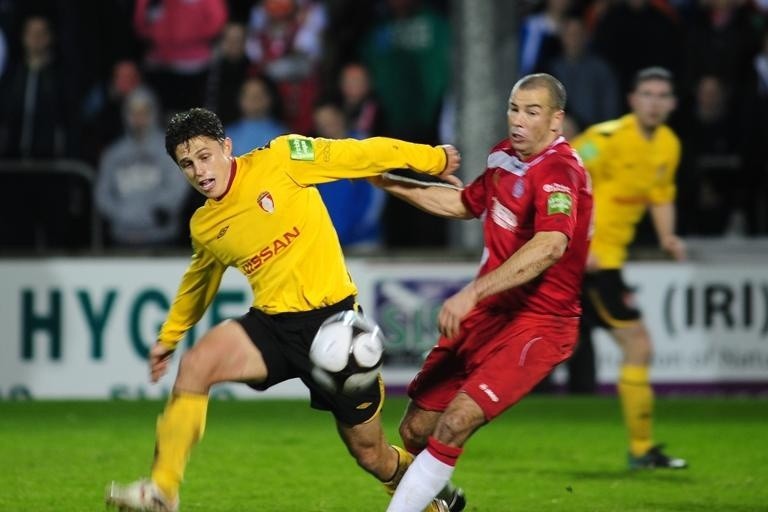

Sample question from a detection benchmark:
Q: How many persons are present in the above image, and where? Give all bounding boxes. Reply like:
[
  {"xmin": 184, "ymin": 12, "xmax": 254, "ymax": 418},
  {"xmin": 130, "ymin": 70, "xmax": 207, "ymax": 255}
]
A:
[
  {"xmin": 348, "ymin": 73, "xmax": 594, "ymax": 512},
  {"xmin": 517, "ymin": 0, "xmax": 767, "ymax": 261},
  {"xmin": 1, "ymin": 2, "xmax": 459, "ymax": 258},
  {"xmin": 101, "ymin": 108, "xmax": 466, "ymax": 512},
  {"xmin": 570, "ymin": 66, "xmax": 689, "ymax": 471}
]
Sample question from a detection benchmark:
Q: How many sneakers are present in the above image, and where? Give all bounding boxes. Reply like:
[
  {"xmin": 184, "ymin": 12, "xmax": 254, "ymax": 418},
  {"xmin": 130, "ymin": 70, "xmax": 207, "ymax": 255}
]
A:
[
  {"xmin": 627, "ymin": 449, "xmax": 687, "ymax": 469},
  {"xmin": 423, "ymin": 488, "xmax": 465, "ymax": 512},
  {"xmin": 105, "ymin": 479, "xmax": 179, "ymax": 512}
]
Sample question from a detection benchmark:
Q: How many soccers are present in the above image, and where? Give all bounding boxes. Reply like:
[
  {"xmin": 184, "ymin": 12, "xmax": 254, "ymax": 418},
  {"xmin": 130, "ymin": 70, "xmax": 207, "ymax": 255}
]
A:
[{"xmin": 309, "ymin": 311, "xmax": 385, "ymax": 392}]
